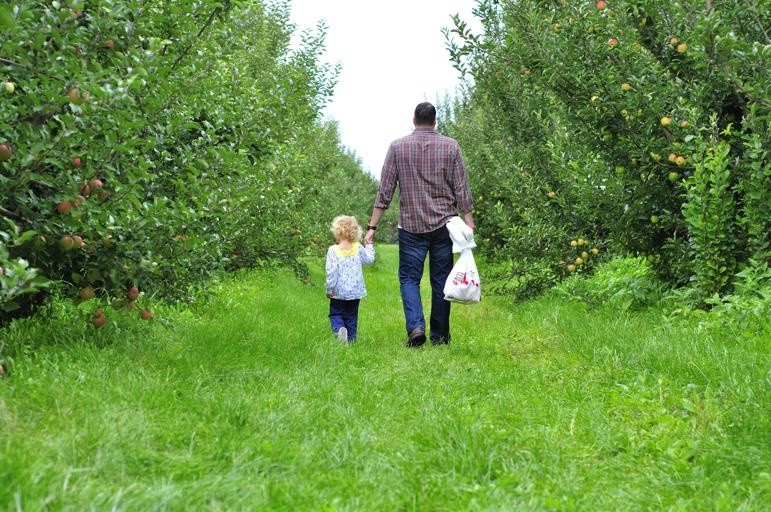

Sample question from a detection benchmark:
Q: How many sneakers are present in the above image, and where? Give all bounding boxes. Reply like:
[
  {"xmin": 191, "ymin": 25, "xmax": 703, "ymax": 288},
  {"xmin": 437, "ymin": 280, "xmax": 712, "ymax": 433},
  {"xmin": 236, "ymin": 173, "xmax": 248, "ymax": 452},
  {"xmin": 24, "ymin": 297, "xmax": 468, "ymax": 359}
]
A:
[
  {"xmin": 406, "ymin": 328, "xmax": 426, "ymax": 347},
  {"xmin": 339, "ymin": 327, "xmax": 348, "ymax": 345}
]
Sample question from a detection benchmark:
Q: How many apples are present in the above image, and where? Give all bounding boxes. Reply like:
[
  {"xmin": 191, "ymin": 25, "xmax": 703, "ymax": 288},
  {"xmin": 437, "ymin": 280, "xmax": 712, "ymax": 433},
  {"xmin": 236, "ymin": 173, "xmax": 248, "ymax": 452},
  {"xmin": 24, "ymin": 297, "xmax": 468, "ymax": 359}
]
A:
[
  {"xmin": 545, "ymin": 2, "xmax": 689, "ymax": 273},
  {"xmin": 1, "ymin": 9, "xmax": 154, "ymax": 326}
]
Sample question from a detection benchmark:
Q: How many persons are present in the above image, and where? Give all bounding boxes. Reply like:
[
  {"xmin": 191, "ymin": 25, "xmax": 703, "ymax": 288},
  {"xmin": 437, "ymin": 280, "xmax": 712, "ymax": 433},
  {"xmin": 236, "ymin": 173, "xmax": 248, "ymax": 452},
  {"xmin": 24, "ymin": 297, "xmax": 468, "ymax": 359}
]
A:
[
  {"xmin": 324, "ymin": 214, "xmax": 377, "ymax": 347},
  {"xmin": 364, "ymin": 101, "xmax": 478, "ymax": 347}
]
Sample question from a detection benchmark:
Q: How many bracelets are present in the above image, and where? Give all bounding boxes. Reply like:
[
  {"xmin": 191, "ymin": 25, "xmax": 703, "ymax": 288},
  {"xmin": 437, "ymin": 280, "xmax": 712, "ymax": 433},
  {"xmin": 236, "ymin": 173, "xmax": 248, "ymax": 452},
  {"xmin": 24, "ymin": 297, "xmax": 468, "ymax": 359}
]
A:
[{"xmin": 368, "ymin": 224, "xmax": 377, "ymax": 231}]
